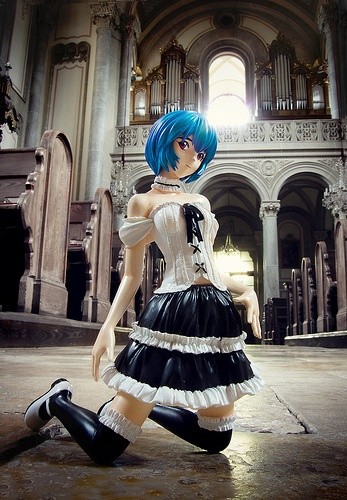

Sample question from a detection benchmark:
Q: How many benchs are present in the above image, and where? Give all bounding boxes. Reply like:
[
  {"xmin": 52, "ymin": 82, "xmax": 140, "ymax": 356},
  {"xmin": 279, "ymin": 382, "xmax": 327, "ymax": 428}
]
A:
[
  {"xmin": 134, "ymin": 244, "xmax": 152, "ymax": 321},
  {"xmin": 261, "ymin": 219, "xmax": 347, "ymax": 348},
  {"xmin": 62, "ymin": 187, "xmax": 112, "ymax": 325},
  {"xmin": 107, "ymin": 228, "xmax": 139, "ymax": 329},
  {"xmin": 0, "ymin": 131, "xmax": 73, "ymax": 316}
]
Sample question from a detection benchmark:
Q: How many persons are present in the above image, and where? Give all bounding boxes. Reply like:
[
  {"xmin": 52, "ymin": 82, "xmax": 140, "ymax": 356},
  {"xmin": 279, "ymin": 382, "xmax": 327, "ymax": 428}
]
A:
[{"xmin": 24, "ymin": 110, "xmax": 261, "ymax": 465}]
